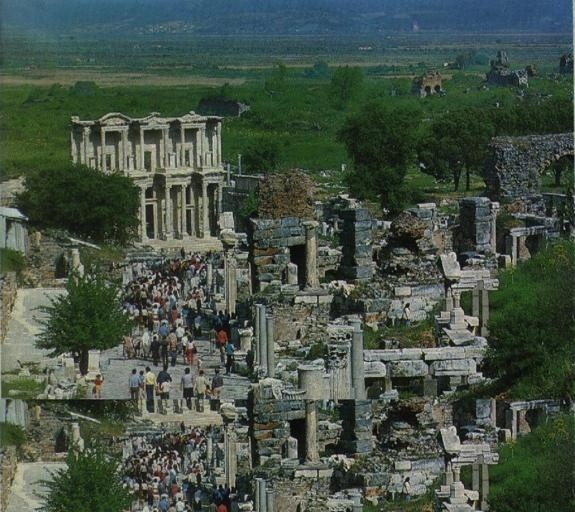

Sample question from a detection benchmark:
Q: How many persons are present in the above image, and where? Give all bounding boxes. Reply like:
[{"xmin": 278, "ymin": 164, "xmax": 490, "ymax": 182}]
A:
[
  {"xmin": 95, "ymin": 374, "xmax": 104, "ymax": 398},
  {"xmin": 110, "ymin": 427, "xmax": 254, "ymax": 512},
  {"xmin": 118, "ymin": 252, "xmax": 238, "ymax": 414}
]
